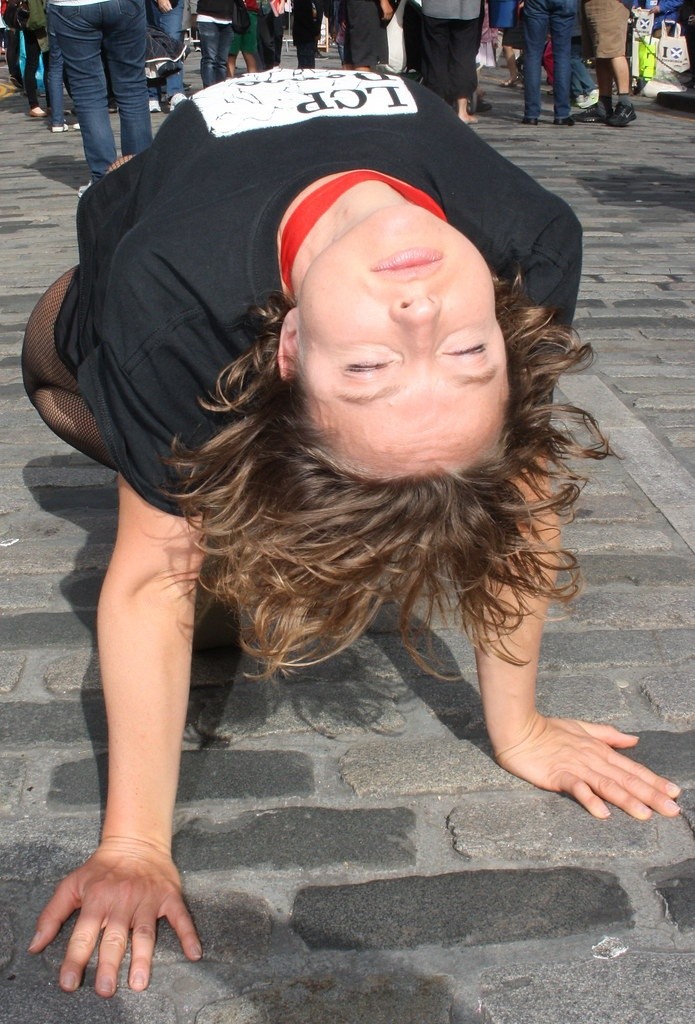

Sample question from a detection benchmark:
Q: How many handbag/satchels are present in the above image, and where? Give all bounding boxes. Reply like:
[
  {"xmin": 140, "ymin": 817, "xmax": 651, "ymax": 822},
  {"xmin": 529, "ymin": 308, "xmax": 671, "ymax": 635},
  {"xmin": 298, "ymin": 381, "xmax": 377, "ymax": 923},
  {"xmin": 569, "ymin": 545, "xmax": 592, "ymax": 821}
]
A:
[{"xmin": 656, "ymin": 20, "xmax": 690, "ymax": 74}]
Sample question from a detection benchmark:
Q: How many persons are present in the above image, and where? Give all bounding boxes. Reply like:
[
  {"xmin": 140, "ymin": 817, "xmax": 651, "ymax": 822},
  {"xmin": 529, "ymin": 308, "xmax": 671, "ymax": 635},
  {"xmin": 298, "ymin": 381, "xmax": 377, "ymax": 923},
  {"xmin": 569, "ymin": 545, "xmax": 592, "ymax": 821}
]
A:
[
  {"xmin": 45, "ymin": 0, "xmax": 158, "ymax": 201},
  {"xmin": 0, "ymin": 1, "xmax": 66, "ymax": 123},
  {"xmin": 421, "ymin": 0, "xmax": 481, "ymax": 126},
  {"xmin": 16, "ymin": 64, "xmax": 681, "ymax": 998},
  {"xmin": 522, "ymin": 0, "xmax": 572, "ymax": 127},
  {"xmin": 139, "ymin": 0, "xmax": 422, "ymax": 109},
  {"xmin": 542, "ymin": 0, "xmax": 601, "ymax": 111},
  {"xmin": 577, "ymin": 1, "xmax": 637, "ymax": 126},
  {"xmin": 647, "ymin": 0, "xmax": 692, "ymax": 93},
  {"xmin": 489, "ymin": 4, "xmax": 531, "ymax": 90}
]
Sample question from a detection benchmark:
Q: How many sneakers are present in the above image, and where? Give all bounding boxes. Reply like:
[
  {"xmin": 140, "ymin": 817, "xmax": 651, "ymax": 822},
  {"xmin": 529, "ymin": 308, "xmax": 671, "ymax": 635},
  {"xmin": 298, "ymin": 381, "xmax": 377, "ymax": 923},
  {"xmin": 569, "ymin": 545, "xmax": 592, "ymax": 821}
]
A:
[
  {"xmin": 499, "ymin": 76, "xmax": 647, "ymax": 126},
  {"xmin": 10, "ymin": 76, "xmax": 187, "ymax": 131},
  {"xmin": 78, "ymin": 181, "xmax": 92, "ymax": 197}
]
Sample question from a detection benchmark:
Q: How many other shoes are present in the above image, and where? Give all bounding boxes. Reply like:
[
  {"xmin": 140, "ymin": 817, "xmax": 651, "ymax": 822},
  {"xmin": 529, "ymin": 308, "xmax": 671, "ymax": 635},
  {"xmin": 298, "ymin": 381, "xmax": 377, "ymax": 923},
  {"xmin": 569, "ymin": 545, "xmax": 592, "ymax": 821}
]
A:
[
  {"xmin": 194, "ymin": 46, "xmax": 201, "ymax": 52},
  {"xmin": 459, "ymin": 62, "xmax": 492, "ymax": 124},
  {"xmin": 315, "ymin": 53, "xmax": 329, "ymax": 59}
]
